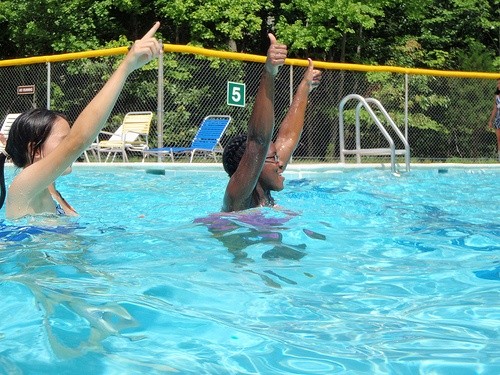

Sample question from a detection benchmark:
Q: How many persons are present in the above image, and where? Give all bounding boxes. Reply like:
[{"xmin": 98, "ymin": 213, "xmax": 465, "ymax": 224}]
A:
[
  {"xmin": 0, "ymin": 21, "xmax": 160, "ymax": 229},
  {"xmin": 220, "ymin": 33, "xmax": 323, "ymax": 213},
  {"xmin": 489, "ymin": 78, "xmax": 500, "ymax": 163}
]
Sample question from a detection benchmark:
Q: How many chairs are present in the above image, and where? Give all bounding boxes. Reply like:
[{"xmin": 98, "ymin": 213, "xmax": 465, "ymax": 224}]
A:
[
  {"xmin": 93, "ymin": 111, "xmax": 153, "ymax": 162},
  {"xmin": 0, "ymin": 113, "xmax": 22, "ymax": 153},
  {"xmin": 141, "ymin": 115, "xmax": 231, "ymax": 163}
]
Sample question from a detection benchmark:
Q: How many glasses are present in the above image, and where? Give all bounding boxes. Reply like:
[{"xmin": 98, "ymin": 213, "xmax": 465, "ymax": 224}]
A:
[{"xmin": 266, "ymin": 155, "xmax": 280, "ymax": 163}]
